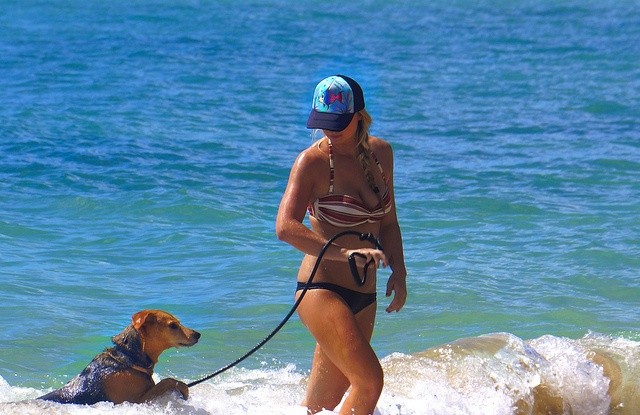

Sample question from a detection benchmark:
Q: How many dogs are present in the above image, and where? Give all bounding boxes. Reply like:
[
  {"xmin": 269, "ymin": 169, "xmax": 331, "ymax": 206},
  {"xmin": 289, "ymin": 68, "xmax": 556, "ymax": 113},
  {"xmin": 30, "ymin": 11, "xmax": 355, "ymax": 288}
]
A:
[{"xmin": 35, "ymin": 308, "xmax": 201, "ymax": 405}]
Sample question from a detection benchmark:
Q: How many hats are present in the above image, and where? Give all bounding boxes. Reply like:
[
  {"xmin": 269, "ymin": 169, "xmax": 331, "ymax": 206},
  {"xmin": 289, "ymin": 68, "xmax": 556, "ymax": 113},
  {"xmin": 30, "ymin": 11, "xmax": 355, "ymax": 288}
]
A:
[{"xmin": 307, "ymin": 75, "xmax": 365, "ymax": 132}]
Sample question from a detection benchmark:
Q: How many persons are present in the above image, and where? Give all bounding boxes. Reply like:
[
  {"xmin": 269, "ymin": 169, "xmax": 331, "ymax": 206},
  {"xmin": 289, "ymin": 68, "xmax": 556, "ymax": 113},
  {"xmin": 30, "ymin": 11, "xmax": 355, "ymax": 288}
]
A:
[{"xmin": 274, "ymin": 74, "xmax": 407, "ymax": 415}]
